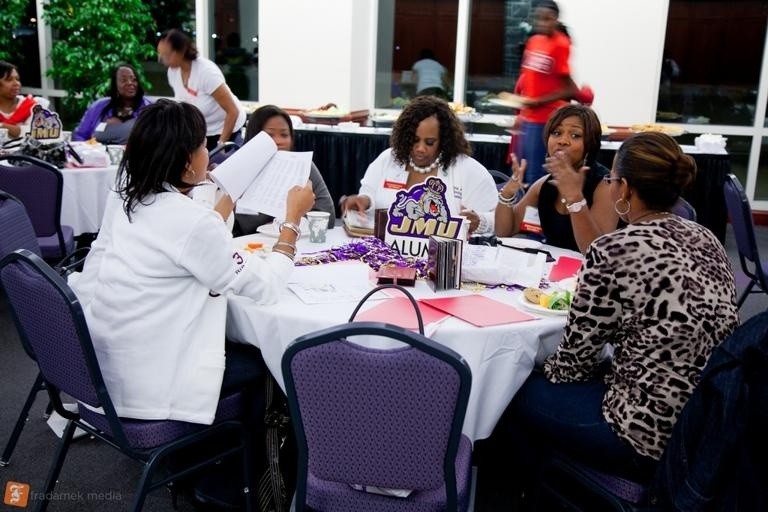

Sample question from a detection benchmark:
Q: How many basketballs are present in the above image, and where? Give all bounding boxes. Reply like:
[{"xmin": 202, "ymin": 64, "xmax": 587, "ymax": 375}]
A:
[
  {"xmin": 524, "ymin": 287, "xmax": 547, "ymax": 303},
  {"xmin": 631, "ymin": 122, "xmax": 671, "ymax": 133}
]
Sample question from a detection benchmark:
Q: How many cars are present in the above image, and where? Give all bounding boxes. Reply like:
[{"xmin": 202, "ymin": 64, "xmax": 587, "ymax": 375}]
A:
[
  {"xmin": 106, "ymin": 145, "xmax": 125, "ymax": 166},
  {"xmin": 306, "ymin": 211, "xmax": 331, "ymax": 243}
]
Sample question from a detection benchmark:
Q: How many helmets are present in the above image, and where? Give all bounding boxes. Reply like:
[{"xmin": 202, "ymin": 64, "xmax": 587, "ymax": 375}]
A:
[
  {"xmin": 256, "ymin": 225, "xmax": 310, "ymax": 239},
  {"xmin": 518, "ymin": 292, "xmax": 573, "ymax": 316},
  {"xmin": 496, "ymin": 238, "xmax": 545, "ymax": 251}
]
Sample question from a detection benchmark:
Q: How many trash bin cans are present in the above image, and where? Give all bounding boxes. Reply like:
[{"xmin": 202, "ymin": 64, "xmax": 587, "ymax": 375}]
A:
[{"xmin": 564, "ymin": 198, "xmax": 588, "ymax": 216}]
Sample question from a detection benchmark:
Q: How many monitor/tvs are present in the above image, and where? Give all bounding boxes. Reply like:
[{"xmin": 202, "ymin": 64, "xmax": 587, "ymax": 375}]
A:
[
  {"xmin": 342, "ymin": 205, "xmax": 379, "ymax": 239},
  {"xmin": 422, "ymin": 295, "xmax": 543, "ymax": 330},
  {"xmin": 349, "ymin": 296, "xmax": 453, "ymax": 334},
  {"xmin": 209, "ymin": 131, "xmax": 314, "ymax": 220}
]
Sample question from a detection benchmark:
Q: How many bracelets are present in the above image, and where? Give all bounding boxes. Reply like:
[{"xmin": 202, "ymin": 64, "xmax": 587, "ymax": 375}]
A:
[
  {"xmin": 694, "ymin": 137, "xmax": 726, "ymax": 153},
  {"xmin": 337, "ymin": 121, "xmax": 361, "ymax": 133},
  {"xmin": 272, "ymin": 214, "xmax": 307, "ymax": 233}
]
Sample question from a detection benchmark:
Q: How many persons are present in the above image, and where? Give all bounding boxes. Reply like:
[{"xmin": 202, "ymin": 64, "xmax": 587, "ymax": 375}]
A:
[
  {"xmin": 341, "ymin": 91, "xmax": 501, "ymax": 239},
  {"xmin": 411, "ymin": 46, "xmax": 452, "ymax": 103},
  {"xmin": 155, "ymin": 27, "xmax": 248, "ymax": 166},
  {"xmin": 70, "ymin": 62, "xmax": 156, "ymax": 145},
  {"xmin": 504, "ymin": 19, "xmax": 596, "ymax": 174},
  {"xmin": 66, "ymin": 94, "xmax": 266, "ymax": 429},
  {"xmin": 1, "ymin": 59, "xmax": 50, "ymax": 139},
  {"xmin": 492, "ymin": 103, "xmax": 627, "ymax": 261},
  {"xmin": 507, "ymin": 129, "xmax": 744, "ymax": 486},
  {"xmin": 513, "ymin": 0, "xmax": 571, "ymax": 195},
  {"xmin": 233, "ymin": 103, "xmax": 337, "ymax": 233}
]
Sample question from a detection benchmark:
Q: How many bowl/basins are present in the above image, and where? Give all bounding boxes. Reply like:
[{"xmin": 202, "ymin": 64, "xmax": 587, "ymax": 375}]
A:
[{"xmin": 628, "ymin": 211, "xmax": 674, "ymax": 221}]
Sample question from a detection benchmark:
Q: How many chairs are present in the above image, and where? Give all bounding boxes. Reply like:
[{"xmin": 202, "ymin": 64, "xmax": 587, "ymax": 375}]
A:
[{"xmin": 0, "ymin": 113, "xmax": 768, "ymax": 512}]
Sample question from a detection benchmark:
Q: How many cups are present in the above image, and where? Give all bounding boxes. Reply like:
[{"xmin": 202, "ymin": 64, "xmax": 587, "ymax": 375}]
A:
[{"xmin": 603, "ymin": 174, "xmax": 621, "ymax": 184}]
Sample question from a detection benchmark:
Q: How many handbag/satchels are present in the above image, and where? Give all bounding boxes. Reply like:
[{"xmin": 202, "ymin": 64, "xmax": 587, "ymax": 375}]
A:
[
  {"xmin": 494, "ymin": 192, "xmax": 518, "ymax": 209},
  {"xmin": 271, "ymin": 222, "xmax": 301, "ymax": 261}
]
